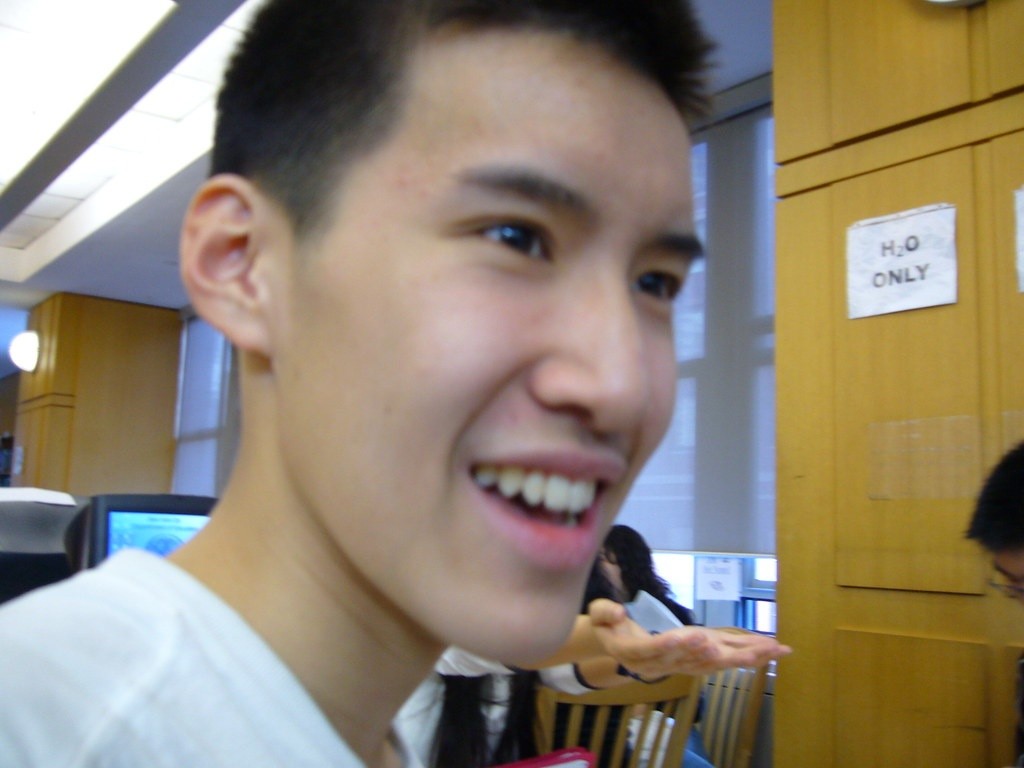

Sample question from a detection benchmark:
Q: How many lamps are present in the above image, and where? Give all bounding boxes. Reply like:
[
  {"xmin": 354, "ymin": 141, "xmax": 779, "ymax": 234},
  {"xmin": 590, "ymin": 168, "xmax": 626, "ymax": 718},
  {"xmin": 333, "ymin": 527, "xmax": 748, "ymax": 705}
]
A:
[{"xmin": 9, "ymin": 329, "xmax": 39, "ymax": 372}]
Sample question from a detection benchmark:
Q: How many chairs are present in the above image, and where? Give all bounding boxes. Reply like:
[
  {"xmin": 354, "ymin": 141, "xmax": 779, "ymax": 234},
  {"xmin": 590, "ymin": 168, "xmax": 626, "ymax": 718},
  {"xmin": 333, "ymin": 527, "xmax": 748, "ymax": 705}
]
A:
[
  {"xmin": 532, "ymin": 675, "xmax": 705, "ymax": 768},
  {"xmin": 692, "ymin": 627, "xmax": 768, "ymax": 768}
]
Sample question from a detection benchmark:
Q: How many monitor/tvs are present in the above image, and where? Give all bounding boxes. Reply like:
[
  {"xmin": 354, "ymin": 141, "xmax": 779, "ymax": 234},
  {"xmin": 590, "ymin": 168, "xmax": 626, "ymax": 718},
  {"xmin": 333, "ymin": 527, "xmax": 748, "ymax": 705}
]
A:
[{"xmin": 81, "ymin": 490, "xmax": 217, "ymax": 570}]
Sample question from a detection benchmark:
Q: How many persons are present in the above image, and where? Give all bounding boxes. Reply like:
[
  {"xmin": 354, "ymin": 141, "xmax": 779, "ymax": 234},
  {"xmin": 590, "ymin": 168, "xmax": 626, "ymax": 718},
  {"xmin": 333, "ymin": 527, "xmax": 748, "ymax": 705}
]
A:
[
  {"xmin": 583, "ymin": 523, "xmax": 707, "ymax": 721},
  {"xmin": 0, "ymin": 0, "xmax": 723, "ymax": 768},
  {"xmin": 388, "ymin": 598, "xmax": 793, "ymax": 768},
  {"xmin": 963, "ymin": 438, "xmax": 1024, "ymax": 768}
]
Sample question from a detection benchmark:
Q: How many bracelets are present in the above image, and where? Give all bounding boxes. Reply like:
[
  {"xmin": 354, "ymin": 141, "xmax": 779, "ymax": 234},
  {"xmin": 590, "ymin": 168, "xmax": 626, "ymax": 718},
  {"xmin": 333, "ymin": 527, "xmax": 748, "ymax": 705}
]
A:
[{"xmin": 616, "ymin": 664, "xmax": 671, "ymax": 685}]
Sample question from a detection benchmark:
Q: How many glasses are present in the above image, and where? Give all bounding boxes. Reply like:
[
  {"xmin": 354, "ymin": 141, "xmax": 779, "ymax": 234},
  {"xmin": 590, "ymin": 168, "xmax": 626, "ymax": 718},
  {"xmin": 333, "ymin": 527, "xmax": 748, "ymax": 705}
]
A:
[{"xmin": 987, "ymin": 567, "xmax": 1024, "ymax": 603}]
